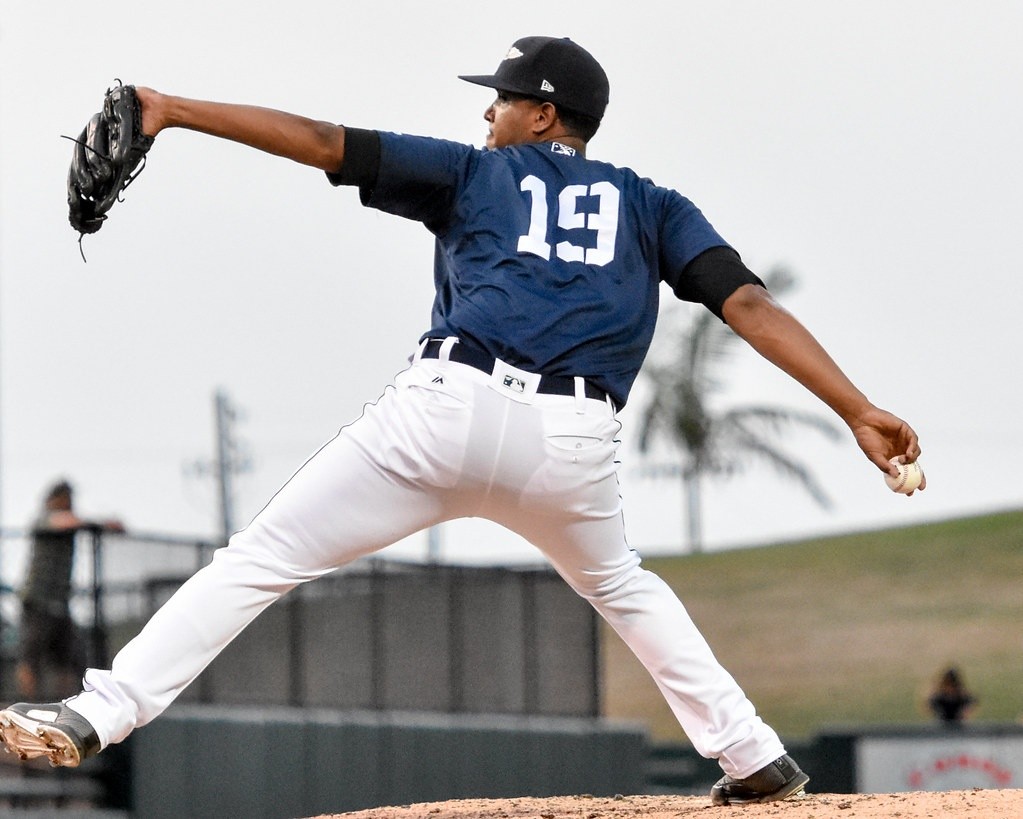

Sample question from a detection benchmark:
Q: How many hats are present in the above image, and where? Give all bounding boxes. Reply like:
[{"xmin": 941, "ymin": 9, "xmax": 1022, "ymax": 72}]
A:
[{"xmin": 456, "ymin": 36, "xmax": 610, "ymax": 123}]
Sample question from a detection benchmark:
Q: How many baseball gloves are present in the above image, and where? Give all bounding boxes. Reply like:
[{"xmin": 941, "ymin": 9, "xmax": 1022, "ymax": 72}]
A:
[{"xmin": 66, "ymin": 83, "xmax": 155, "ymax": 235}]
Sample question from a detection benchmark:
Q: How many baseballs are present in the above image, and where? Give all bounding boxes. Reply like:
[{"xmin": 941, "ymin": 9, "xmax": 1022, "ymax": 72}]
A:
[{"xmin": 884, "ymin": 453, "xmax": 923, "ymax": 494}]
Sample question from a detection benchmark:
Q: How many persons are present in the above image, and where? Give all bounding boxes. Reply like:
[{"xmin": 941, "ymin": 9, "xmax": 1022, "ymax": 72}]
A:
[
  {"xmin": 15, "ymin": 482, "xmax": 125, "ymax": 700},
  {"xmin": 0, "ymin": 36, "xmax": 926, "ymax": 803},
  {"xmin": 929, "ymin": 671, "xmax": 974, "ymax": 728}
]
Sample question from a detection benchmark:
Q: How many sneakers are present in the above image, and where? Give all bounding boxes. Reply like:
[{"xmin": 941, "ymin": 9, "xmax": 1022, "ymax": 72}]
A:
[
  {"xmin": 710, "ymin": 754, "xmax": 810, "ymax": 806},
  {"xmin": 0, "ymin": 694, "xmax": 101, "ymax": 769}
]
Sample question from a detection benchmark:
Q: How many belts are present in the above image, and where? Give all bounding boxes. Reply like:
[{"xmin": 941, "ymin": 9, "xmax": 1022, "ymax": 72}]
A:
[{"xmin": 421, "ymin": 340, "xmax": 615, "ymax": 409}]
